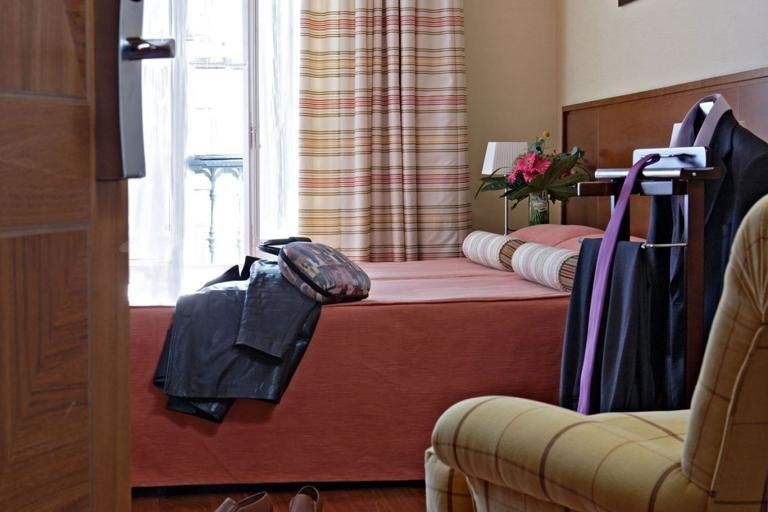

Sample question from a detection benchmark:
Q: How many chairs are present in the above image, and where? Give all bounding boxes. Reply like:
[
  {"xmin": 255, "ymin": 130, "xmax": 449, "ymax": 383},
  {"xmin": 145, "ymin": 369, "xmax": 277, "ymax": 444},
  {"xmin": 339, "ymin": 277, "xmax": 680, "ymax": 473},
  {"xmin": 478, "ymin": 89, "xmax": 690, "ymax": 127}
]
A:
[{"xmin": 424, "ymin": 193, "xmax": 767, "ymax": 512}]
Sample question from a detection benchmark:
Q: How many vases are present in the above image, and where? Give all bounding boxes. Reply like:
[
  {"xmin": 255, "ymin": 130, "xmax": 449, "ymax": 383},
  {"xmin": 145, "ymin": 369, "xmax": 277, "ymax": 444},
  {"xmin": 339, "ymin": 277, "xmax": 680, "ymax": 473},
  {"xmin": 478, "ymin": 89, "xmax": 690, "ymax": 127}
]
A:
[{"xmin": 529, "ymin": 193, "xmax": 550, "ymax": 225}]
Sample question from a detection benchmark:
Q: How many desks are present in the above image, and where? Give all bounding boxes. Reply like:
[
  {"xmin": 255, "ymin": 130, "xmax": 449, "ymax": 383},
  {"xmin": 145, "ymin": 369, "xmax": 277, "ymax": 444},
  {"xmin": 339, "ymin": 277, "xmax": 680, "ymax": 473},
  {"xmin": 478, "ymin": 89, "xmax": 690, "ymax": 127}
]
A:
[{"xmin": 186, "ymin": 153, "xmax": 244, "ymax": 263}]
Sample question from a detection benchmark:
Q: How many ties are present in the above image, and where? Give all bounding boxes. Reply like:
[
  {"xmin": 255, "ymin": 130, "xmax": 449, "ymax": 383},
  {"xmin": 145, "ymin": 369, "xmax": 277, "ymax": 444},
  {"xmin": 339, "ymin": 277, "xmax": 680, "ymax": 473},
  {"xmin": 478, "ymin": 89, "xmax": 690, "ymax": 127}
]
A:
[{"xmin": 578, "ymin": 153, "xmax": 661, "ymax": 415}]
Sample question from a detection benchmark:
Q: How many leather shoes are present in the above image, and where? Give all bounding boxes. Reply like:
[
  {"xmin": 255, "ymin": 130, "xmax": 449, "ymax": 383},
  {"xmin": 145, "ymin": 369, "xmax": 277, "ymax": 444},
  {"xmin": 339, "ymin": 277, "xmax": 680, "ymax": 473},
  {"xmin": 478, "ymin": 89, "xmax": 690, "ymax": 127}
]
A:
[
  {"xmin": 288, "ymin": 486, "xmax": 321, "ymax": 512},
  {"xmin": 215, "ymin": 492, "xmax": 272, "ymax": 512}
]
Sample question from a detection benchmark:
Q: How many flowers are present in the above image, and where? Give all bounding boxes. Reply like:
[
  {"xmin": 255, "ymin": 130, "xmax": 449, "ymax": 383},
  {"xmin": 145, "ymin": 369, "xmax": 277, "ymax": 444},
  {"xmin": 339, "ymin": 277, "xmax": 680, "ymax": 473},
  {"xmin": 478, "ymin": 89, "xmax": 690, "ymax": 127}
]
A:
[{"xmin": 474, "ymin": 129, "xmax": 594, "ymax": 224}]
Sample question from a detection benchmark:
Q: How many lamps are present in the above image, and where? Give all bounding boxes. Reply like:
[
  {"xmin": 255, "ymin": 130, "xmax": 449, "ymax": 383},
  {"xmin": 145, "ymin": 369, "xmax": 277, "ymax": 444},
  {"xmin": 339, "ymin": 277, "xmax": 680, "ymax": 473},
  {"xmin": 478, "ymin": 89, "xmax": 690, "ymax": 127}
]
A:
[{"xmin": 480, "ymin": 141, "xmax": 528, "ymax": 235}]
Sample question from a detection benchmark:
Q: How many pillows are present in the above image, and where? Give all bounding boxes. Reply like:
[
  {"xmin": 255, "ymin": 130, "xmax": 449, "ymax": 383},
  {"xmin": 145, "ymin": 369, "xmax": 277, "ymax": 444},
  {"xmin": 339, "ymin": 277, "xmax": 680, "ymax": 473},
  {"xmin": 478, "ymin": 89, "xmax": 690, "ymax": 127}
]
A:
[{"xmin": 461, "ymin": 222, "xmax": 648, "ymax": 293}]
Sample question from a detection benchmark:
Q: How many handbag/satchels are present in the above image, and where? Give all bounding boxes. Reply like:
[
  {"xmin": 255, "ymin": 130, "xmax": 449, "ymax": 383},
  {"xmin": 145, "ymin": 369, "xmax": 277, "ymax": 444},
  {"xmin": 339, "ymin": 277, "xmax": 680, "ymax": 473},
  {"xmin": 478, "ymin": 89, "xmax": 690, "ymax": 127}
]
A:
[{"xmin": 278, "ymin": 241, "xmax": 372, "ymax": 303}]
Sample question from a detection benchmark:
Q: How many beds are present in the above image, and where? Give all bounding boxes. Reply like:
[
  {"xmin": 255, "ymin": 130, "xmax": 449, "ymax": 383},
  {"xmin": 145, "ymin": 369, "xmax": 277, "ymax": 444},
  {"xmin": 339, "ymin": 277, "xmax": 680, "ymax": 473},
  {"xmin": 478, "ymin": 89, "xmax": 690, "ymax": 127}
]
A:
[{"xmin": 127, "ymin": 67, "xmax": 767, "ymax": 490}]
